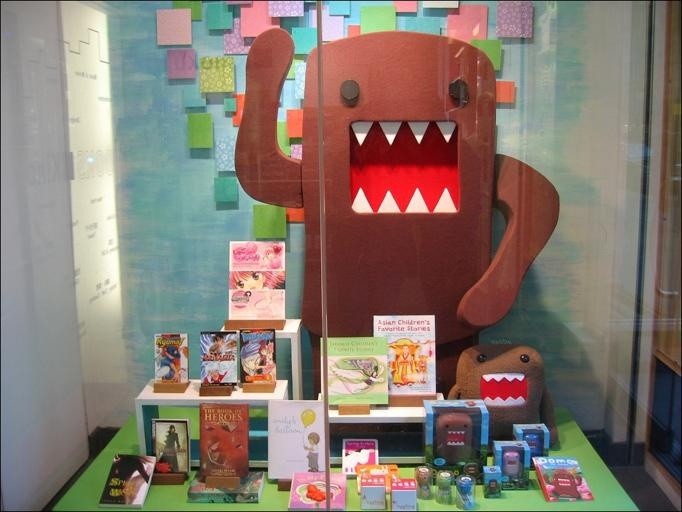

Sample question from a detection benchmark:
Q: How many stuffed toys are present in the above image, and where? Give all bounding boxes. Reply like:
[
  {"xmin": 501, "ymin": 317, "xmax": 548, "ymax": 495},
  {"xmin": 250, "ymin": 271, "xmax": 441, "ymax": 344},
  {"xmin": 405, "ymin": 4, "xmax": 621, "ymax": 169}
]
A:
[
  {"xmin": 445, "ymin": 341, "xmax": 560, "ymax": 448},
  {"xmin": 421, "ymin": 399, "xmax": 491, "ymax": 484},
  {"xmin": 494, "ymin": 440, "xmax": 530, "ymax": 493},
  {"xmin": 511, "ymin": 420, "xmax": 552, "ymax": 459}
]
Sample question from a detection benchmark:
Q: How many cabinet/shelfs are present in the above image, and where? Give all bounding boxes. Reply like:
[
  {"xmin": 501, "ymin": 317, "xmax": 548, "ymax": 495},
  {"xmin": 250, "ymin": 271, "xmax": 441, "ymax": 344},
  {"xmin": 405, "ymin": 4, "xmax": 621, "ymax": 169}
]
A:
[{"xmin": 40, "ymin": 318, "xmax": 638, "ymax": 511}]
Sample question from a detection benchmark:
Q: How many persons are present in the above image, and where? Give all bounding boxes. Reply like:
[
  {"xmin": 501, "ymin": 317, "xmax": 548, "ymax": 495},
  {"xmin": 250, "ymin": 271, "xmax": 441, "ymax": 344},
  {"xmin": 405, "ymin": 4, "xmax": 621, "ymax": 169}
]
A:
[
  {"xmin": 303, "ymin": 432, "xmax": 320, "ymax": 472},
  {"xmin": 390, "ymin": 344, "xmax": 419, "ymax": 388},
  {"xmin": 157, "ymin": 426, "xmax": 182, "ymax": 472}
]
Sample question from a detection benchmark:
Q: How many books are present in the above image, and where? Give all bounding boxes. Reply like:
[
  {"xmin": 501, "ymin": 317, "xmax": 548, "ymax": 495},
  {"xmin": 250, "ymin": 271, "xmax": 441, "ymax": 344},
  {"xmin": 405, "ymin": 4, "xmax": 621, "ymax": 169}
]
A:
[
  {"xmin": 235, "ymin": 328, "xmax": 277, "ymax": 384},
  {"xmin": 186, "ymin": 470, "xmax": 265, "ymax": 503},
  {"xmin": 199, "ymin": 402, "xmax": 249, "ymax": 482},
  {"xmin": 532, "ymin": 455, "xmax": 595, "ymax": 503},
  {"xmin": 286, "ymin": 469, "xmax": 346, "ymax": 511},
  {"xmin": 98, "ymin": 453, "xmax": 156, "ymax": 510},
  {"xmin": 356, "ymin": 463, "xmax": 401, "ymax": 496},
  {"xmin": 224, "ymin": 240, "xmax": 288, "ymax": 322},
  {"xmin": 150, "ymin": 417, "xmax": 190, "ymax": 475},
  {"xmin": 317, "ymin": 336, "xmax": 389, "ymax": 406},
  {"xmin": 148, "ymin": 328, "xmax": 191, "ymax": 386},
  {"xmin": 195, "ymin": 327, "xmax": 241, "ymax": 387},
  {"xmin": 342, "ymin": 436, "xmax": 379, "ymax": 479},
  {"xmin": 264, "ymin": 399, "xmax": 325, "ymax": 481},
  {"xmin": 373, "ymin": 313, "xmax": 438, "ymax": 396}
]
